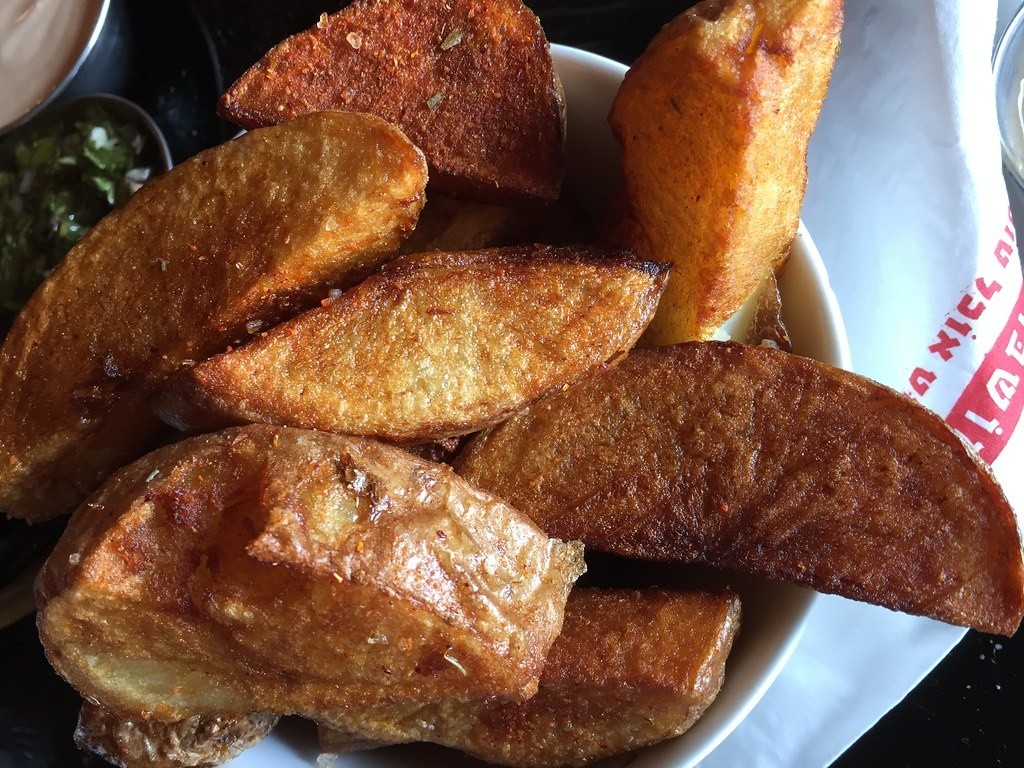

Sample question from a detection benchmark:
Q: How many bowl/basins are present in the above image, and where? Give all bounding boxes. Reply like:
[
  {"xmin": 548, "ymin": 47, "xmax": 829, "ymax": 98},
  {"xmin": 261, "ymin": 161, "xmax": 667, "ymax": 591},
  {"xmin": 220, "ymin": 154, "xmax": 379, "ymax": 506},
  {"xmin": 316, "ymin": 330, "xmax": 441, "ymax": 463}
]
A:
[{"xmin": 75, "ymin": 45, "xmax": 854, "ymax": 768}]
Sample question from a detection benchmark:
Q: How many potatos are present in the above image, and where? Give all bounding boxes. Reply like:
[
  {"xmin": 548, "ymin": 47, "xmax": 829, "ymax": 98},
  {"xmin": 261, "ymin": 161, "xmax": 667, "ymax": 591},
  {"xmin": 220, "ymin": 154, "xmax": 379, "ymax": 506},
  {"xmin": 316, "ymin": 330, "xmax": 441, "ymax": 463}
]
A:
[{"xmin": 1, "ymin": 0, "xmax": 1021, "ymax": 768}]
